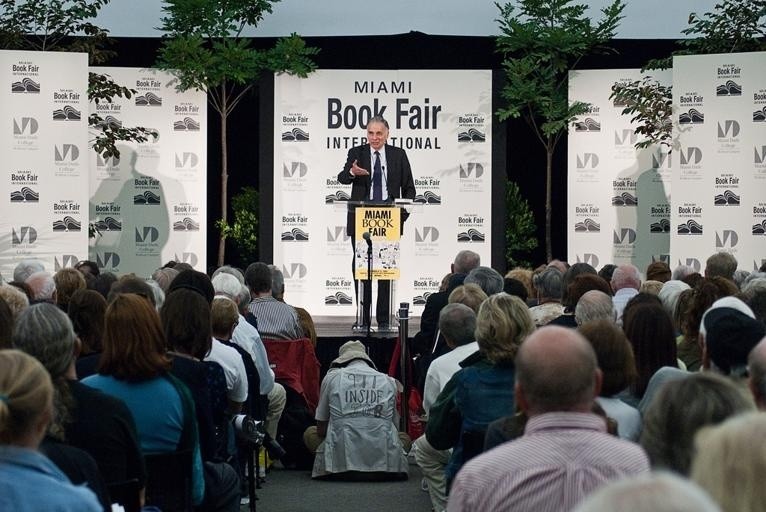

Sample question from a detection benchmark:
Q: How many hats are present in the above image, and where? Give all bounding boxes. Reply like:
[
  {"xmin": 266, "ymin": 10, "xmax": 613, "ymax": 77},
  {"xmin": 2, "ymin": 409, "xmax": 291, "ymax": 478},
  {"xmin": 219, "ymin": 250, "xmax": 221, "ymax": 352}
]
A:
[
  {"xmin": 704, "ymin": 307, "xmax": 766, "ymax": 374},
  {"xmin": 330, "ymin": 341, "xmax": 377, "ymax": 370}
]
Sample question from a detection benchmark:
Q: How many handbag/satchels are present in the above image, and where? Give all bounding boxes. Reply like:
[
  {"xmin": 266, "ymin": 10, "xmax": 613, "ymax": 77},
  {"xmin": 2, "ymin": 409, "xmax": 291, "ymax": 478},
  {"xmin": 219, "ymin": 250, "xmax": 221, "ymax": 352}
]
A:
[{"xmin": 395, "ymin": 385, "xmax": 422, "ymax": 440}]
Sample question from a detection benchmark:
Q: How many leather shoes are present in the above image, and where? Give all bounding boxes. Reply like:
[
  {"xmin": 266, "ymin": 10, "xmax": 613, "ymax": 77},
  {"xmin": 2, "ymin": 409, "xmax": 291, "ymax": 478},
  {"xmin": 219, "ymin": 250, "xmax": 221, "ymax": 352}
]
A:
[{"xmin": 266, "ymin": 440, "xmax": 285, "ymax": 460}]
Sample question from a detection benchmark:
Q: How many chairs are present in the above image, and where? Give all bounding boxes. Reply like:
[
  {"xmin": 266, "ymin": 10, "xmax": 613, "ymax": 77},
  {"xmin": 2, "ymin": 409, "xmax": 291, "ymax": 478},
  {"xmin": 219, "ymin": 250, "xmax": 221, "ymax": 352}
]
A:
[
  {"xmin": 89, "ymin": 331, "xmax": 314, "ymax": 510},
  {"xmin": 485, "ymin": 411, "xmax": 619, "ymax": 447}
]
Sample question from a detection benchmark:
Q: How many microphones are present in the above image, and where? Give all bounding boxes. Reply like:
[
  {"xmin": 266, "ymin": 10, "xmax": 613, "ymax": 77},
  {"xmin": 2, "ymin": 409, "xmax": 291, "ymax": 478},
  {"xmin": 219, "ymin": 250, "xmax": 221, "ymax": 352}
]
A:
[
  {"xmin": 382, "ymin": 166, "xmax": 394, "ymax": 203},
  {"xmin": 363, "ymin": 232, "xmax": 372, "ymax": 247}
]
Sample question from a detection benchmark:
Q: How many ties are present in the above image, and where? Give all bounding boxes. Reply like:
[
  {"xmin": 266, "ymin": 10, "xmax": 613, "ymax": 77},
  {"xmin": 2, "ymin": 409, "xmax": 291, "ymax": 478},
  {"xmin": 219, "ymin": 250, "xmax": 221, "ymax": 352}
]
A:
[{"xmin": 373, "ymin": 151, "xmax": 381, "ymax": 200}]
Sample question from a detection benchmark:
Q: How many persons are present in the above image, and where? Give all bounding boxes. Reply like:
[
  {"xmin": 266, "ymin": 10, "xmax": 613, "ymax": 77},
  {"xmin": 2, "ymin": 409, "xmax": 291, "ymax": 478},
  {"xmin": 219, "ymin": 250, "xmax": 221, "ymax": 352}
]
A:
[
  {"xmin": 0, "ymin": 258, "xmax": 306, "ymax": 512},
  {"xmin": 337, "ymin": 114, "xmax": 416, "ymax": 334},
  {"xmin": 304, "ymin": 340, "xmax": 410, "ymax": 478},
  {"xmin": 411, "ymin": 250, "xmax": 766, "ymax": 512}
]
